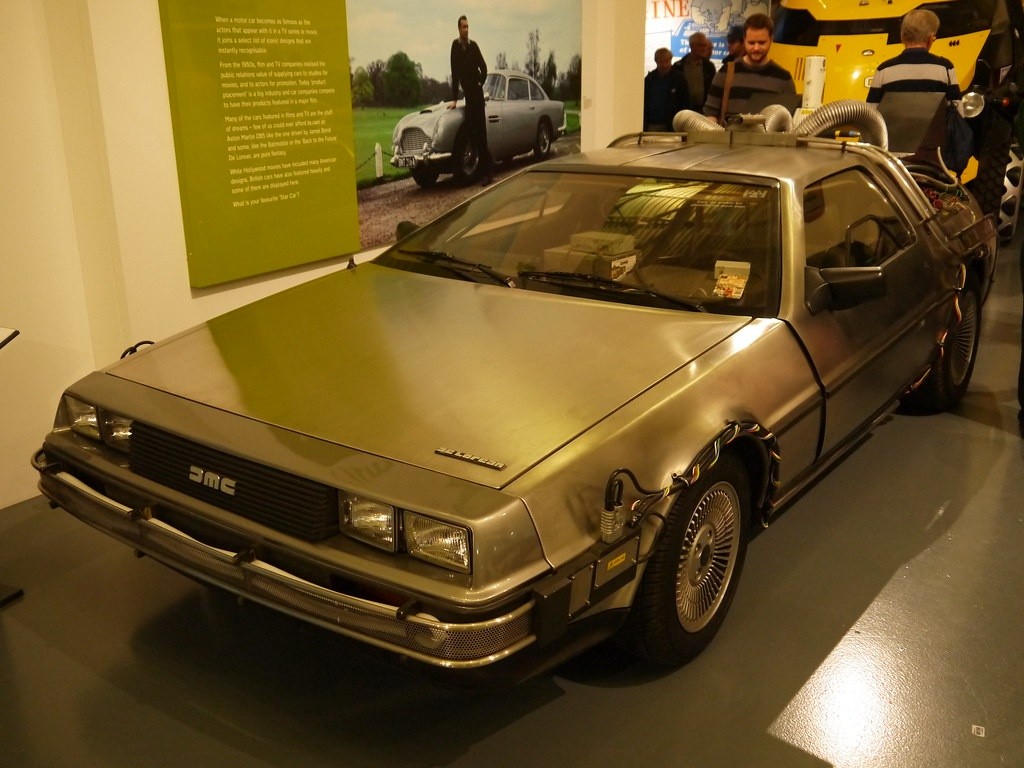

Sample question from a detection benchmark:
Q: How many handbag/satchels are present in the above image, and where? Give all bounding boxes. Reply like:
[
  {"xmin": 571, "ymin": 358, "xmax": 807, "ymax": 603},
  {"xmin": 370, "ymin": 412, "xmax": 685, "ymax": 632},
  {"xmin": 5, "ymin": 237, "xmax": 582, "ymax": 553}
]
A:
[{"xmin": 944, "ymin": 101, "xmax": 972, "ymax": 146}]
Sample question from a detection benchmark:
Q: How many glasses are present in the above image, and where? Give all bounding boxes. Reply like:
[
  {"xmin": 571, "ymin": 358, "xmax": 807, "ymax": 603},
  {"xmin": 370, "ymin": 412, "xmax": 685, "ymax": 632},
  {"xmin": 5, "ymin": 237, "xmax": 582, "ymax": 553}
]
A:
[{"xmin": 932, "ymin": 32, "xmax": 936, "ymax": 42}]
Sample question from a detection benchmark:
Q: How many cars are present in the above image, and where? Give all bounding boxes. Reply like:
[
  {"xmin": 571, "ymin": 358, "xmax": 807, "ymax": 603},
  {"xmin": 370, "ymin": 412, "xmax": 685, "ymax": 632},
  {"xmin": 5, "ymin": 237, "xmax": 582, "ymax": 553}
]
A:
[
  {"xmin": 30, "ymin": 101, "xmax": 1001, "ymax": 692},
  {"xmin": 389, "ymin": 70, "xmax": 568, "ymax": 186}
]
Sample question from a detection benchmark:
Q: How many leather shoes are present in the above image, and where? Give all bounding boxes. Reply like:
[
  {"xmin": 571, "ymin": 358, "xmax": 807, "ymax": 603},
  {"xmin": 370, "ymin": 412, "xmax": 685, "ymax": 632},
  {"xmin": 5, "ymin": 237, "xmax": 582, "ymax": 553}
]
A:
[{"xmin": 481, "ymin": 158, "xmax": 497, "ymax": 188}]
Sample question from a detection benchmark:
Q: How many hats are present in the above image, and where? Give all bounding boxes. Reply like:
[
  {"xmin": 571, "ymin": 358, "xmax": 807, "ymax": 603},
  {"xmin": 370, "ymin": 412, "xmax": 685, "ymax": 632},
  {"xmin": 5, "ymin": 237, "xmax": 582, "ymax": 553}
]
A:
[{"xmin": 727, "ymin": 27, "xmax": 742, "ymax": 38}]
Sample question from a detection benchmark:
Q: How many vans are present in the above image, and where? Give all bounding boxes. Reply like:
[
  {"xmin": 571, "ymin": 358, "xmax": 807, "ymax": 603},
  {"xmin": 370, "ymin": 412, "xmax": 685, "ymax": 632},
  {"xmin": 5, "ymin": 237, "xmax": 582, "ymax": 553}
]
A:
[{"xmin": 764, "ymin": 0, "xmax": 995, "ymax": 186}]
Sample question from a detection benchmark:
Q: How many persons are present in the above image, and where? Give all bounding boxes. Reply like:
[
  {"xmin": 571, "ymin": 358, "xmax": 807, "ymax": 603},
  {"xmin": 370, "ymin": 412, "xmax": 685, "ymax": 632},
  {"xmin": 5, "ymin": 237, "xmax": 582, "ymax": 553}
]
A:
[
  {"xmin": 447, "ymin": 15, "xmax": 497, "ymax": 186},
  {"xmin": 643, "ymin": 49, "xmax": 690, "ymax": 132},
  {"xmin": 673, "ymin": 32, "xmax": 717, "ymax": 115},
  {"xmin": 703, "ymin": 13, "xmax": 797, "ymax": 133},
  {"xmin": 703, "ymin": 26, "xmax": 747, "ymax": 116},
  {"xmin": 865, "ymin": 10, "xmax": 965, "ymax": 154}
]
